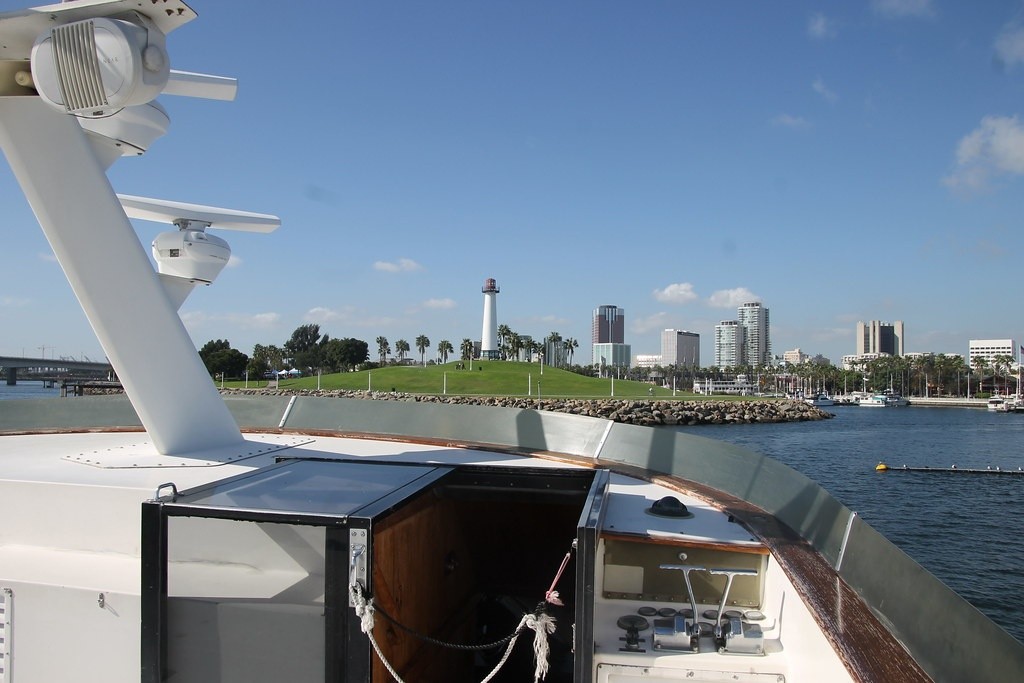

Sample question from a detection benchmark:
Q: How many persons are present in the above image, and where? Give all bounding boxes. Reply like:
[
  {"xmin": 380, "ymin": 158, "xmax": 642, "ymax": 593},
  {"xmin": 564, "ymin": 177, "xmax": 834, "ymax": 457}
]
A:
[{"xmin": 456, "ymin": 362, "xmax": 466, "ymax": 370}]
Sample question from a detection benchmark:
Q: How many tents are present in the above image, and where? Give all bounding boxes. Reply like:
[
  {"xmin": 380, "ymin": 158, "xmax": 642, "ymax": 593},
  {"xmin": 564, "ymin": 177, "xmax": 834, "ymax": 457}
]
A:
[{"xmin": 272, "ymin": 368, "xmax": 303, "ymax": 379}]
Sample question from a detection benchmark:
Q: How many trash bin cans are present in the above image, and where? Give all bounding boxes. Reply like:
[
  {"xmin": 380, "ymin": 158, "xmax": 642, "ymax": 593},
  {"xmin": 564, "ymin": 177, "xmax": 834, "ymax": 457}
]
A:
[
  {"xmin": 392, "ymin": 388, "xmax": 395, "ymax": 392},
  {"xmin": 479, "ymin": 366, "xmax": 483, "ymax": 371}
]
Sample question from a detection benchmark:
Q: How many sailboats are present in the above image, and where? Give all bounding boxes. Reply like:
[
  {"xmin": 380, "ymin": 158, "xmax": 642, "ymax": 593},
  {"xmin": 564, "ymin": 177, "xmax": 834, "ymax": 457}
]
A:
[
  {"xmin": 986, "ymin": 344, "xmax": 1024, "ymax": 412},
  {"xmin": 754, "ymin": 371, "xmax": 911, "ymax": 408}
]
